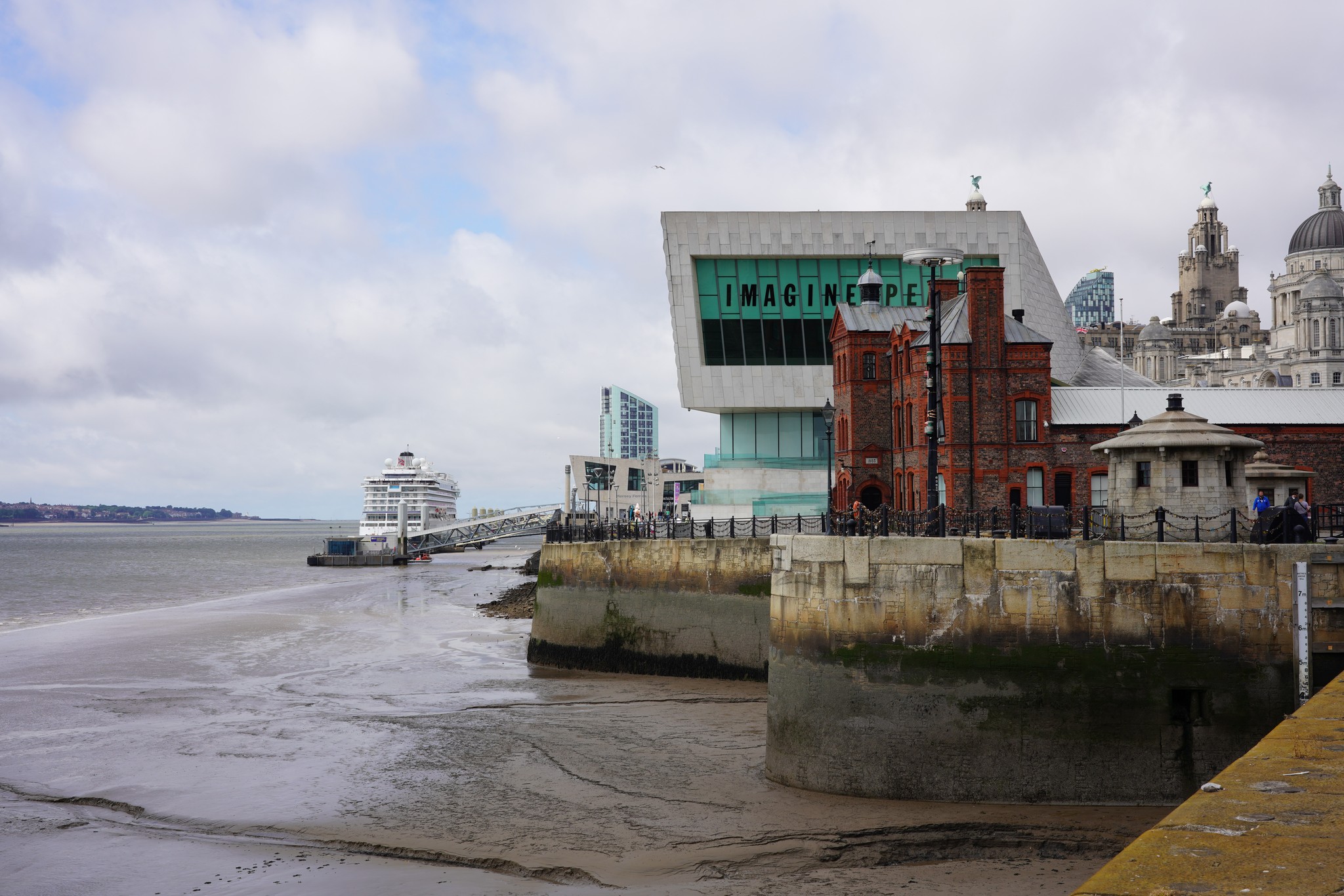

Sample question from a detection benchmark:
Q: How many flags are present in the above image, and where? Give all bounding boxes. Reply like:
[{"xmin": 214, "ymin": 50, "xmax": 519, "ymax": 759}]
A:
[
  {"xmin": 432, "ymin": 462, "xmax": 433, "ymax": 465},
  {"xmin": 398, "ymin": 458, "xmax": 405, "ymax": 466},
  {"xmin": 1075, "ymin": 326, "xmax": 1088, "ymax": 334}
]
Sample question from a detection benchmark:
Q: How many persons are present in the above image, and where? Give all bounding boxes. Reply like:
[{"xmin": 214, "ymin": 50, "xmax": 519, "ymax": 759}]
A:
[
  {"xmin": 639, "ymin": 518, "xmax": 646, "ymax": 537},
  {"xmin": 623, "ymin": 512, "xmax": 626, "ymax": 522},
  {"xmin": 605, "ymin": 515, "xmax": 608, "ymax": 526},
  {"xmin": 1283, "ymin": 491, "xmax": 1300, "ymax": 509},
  {"xmin": 665, "ymin": 509, "xmax": 671, "ymax": 521},
  {"xmin": 621, "ymin": 519, "xmax": 635, "ymax": 534},
  {"xmin": 652, "ymin": 511, "xmax": 656, "ymax": 520},
  {"xmin": 1252, "ymin": 491, "xmax": 1270, "ymax": 517},
  {"xmin": 658, "ymin": 510, "xmax": 662, "ymax": 522},
  {"xmin": 555, "ymin": 521, "xmax": 562, "ymax": 541},
  {"xmin": 650, "ymin": 520, "xmax": 657, "ymax": 538},
  {"xmin": 583, "ymin": 522, "xmax": 599, "ymax": 540},
  {"xmin": 1295, "ymin": 493, "xmax": 1310, "ymax": 523},
  {"xmin": 688, "ymin": 510, "xmax": 690, "ymax": 520},
  {"xmin": 646, "ymin": 510, "xmax": 651, "ymax": 521},
  {"xmin": 685, "ymin": 518, "xmax": 690, "ymax": 523},
  {"xmin": 674, "ymin": 512, "xmax": 678, "ymax": 521}
]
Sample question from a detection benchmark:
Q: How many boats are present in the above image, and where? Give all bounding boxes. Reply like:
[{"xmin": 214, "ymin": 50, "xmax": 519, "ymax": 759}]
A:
[{"xmin": 357, "ymin": 444, "xmax": 461, "ymax": 548}]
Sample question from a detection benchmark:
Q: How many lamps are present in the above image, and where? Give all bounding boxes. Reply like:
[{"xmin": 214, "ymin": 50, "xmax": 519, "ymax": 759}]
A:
[
  {"xmin": 840, "ymin": 463, "xmax": 861, "ymax": 476},
  {"xmin": 1080, "ymin": 434, "xmax": 1086, "ymax": 437}
]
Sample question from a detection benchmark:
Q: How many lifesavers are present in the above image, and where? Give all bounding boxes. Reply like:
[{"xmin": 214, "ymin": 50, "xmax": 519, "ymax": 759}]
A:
[{"xmin": 853, "ymin": 501, "xmax": 860, "ymax": 520}]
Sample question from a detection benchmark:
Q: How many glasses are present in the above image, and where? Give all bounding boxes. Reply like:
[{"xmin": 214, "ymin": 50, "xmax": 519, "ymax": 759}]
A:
[{"xmin": 1295, "ymin": 495, "xmax": 1298, "ymax": 496}]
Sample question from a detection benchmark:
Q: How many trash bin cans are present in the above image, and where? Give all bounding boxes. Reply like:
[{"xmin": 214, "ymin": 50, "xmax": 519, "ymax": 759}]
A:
[
  {"xmin": 1028, "ymin": 506, "xmax": 1066, "ymax": 539},
  {"xmin": 1249, "ymin": 506, "xmax": 1312, "ymax": 544}
]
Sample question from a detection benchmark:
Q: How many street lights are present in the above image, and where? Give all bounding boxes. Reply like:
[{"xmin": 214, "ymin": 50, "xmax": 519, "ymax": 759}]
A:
[
  {"xmin": 582, "ymin": 482, "xmax": 589, "ymax": 535},
  {"xmin": 570, "ymin": 488, "xmax": 578, "ymax": 529},
  {"xmin": 585, "ymin": 474, "xmax": 594, "ymax": 526},
  {"xmin": 613, "ymin": 484, "xmax": 620, "ymax": 525},
  {"xmin": 820, "ymin": 397, "xmax": 838, "ymax": 536},
  {"xmin": 640, "ymin": 483, "xmax": 647, "ymax": 523},
  {"xmin": 592, "ymin": 468, "xmax": 603, "ymax": 527}
]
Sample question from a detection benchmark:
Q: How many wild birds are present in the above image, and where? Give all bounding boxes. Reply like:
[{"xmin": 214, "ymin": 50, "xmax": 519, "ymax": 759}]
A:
[
  {"xmin": 1200, "ymin": 182, "xmax": 1212, "ymax": 198},
  {"xmin": 970, "ymin": 175, "xmax": 981, "ymax": 192},
  {"xmin": 651, "ymin": 166, "xmax": 666, "ymax": 169},
  {"xmin": 515, "ymin": 545, "xmax": 517, "ymax": 548},
  {"xmin": 518, "ymin": 547, "xmax": 520, "ymax": 550},
  {"xmin": 473, "ymin": 613, "xmax": 475, "ymax": 617},
  {"xmin": 444, "ymin": 583, "xmax": 446, "ymax": 586},
  {"xmin": 525, "ymin": 551, "xmax": 528, "ymax": 552}
]
